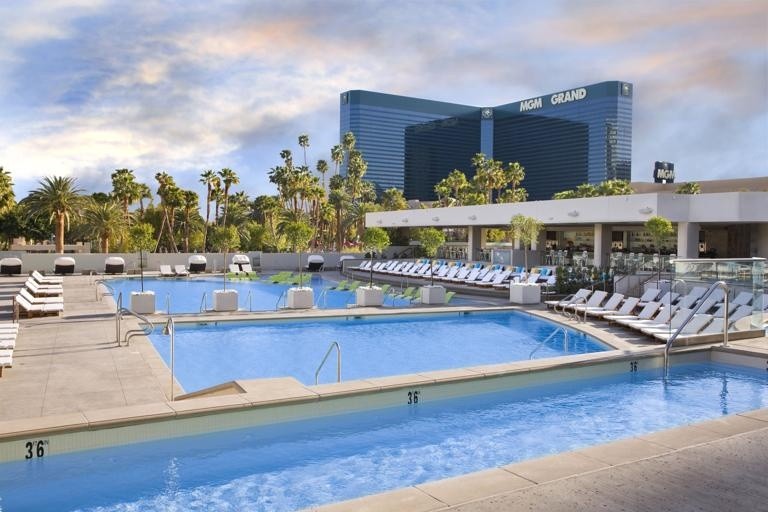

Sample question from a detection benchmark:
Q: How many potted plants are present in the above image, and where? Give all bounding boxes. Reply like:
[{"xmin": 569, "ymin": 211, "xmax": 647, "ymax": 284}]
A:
[
  {"xmin": 418, "ymin": 227, "xmax": 448, "ymax": 305},
  {"xmin": 507, "ymin": 214, "xmax": 544, "ymax": 303},
  {"xmin": 644, "ymin": 215, "xmax": 673, "ymax": 298},
  {"xmin": 207, "ymin": 224, "xmax": 242, "ymax": 313},
  {"xmin": 124, "ymin": 222, "xmax": 157, "ymax": 316},
  {"xmin": 355, "ymin": 225, "xmax": 392, "ymax": 307},
  {"xmin": 279, "ymin": 222, "xmax": 316, "ymax": 310}
]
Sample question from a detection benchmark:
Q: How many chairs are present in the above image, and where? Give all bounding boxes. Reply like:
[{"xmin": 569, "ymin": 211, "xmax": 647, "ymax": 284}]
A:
[
  {"xmin": 158, "ymin": 254, "xmax": 207, "ymax": 278},
  {"xmin": 302, "ymin": 254, "xmax": 355, "ymax": 274},
  {"xmin": 348, "ymin": 252, "xmax": 560, "ymax": 292},
  {"xmin": 0, "ymin": 257, "xmax": 22, "ymax": 276},
  {"xmin": 544, "ymin": 248, "xmax": 676, "ymax": 272},
  {"xmin": 542, "ymin": 283, "xmax": 768, "ymax": 341},
  {"xmin": 0, "ymin": 269, "xmax": 63, "ymax": 379},
  {"xmin": 54, "ymin": 256, "xmax": 75, "ymax": 275},
  {"xmin": 104, "ymin": 256, "xmax": 125, "ymax": 275}
]
